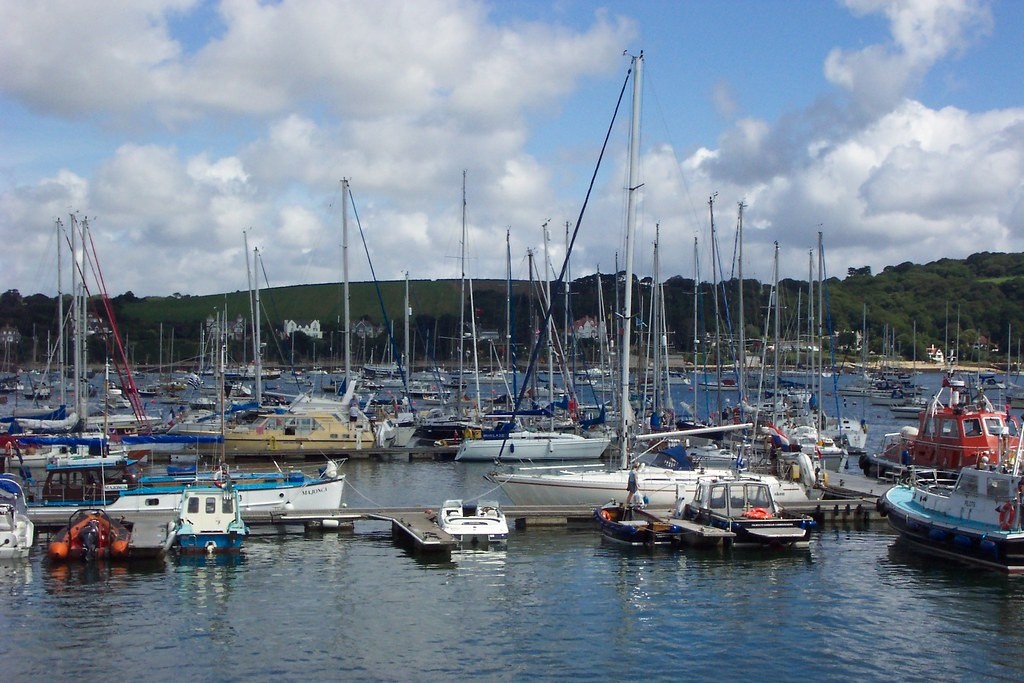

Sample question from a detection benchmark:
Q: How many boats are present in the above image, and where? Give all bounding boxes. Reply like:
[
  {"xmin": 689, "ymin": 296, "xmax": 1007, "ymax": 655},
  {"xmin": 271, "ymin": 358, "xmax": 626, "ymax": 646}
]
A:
[
  {"xmin": 593, "ymin": 498, "xmax": 682, "ymax": 546},
  {"xmin": 436, "ymin": 499, "xmax": 511, "ymax": 545},
  {"xmin": 0, "ymin": 472, "xmax": 35, "ymax": 558},
  {"xmin": 172, "ymin": 435, "xmax": 251, "ymax": 553},
  {"xmin": 48, "ymin": 508, "xmax": 130, "ymax": 560},
  {"xmin": 874, "ymin": 463, "xmax": 1024, "ymax": 585},
  {"xmin": 685, "ymin": 472, "xmax": 819, "ymax": 550}
]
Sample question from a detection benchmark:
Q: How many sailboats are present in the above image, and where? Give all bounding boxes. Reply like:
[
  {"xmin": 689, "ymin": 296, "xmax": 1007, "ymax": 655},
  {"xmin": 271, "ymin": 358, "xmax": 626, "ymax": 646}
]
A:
[{"xmin": 0, "ymin": 49, "xmax": 1024, "ymax": 526}]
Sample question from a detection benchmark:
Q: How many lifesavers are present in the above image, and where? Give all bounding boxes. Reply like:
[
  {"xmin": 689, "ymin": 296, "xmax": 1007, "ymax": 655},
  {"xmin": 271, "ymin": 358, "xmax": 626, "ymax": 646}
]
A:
[
  {"xmin": 214, "ymin": 471, "xmax": 232, "ymax": 489},
  {"xmin": 747, "ymin": 507, "xmax": 771, "ymax": 519},
  {"xmin": 602, "ymin": 510, "xmax": 611, "ymax": 520},
  {"xmin": 999, "ymin": 503, "xmax": 1016, "ymax": 530}
]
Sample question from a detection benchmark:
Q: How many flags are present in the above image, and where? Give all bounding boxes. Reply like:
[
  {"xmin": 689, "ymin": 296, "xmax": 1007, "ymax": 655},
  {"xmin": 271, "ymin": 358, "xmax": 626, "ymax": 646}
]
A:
[
  {"xmin": 767, "ymin": 424, "xmax": 788, "ymax": 445},
  {"xmin": 187, "ymin": 373, "xmax": 200, "ymax": 390}
]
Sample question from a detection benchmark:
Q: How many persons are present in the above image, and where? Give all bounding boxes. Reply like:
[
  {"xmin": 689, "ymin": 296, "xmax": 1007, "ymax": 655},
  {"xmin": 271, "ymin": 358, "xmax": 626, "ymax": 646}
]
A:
[
  {"xmin": 722, "ymin": 409, "xmax": 728, "ymax": 427},
  {"xmin": 626, "ymin": 465, "xmax": 641, "ymax": 508},
  {"xmin": 349, "ymin": 393, "xmax": 359, "ymax": 431},
  {"xmin": 85, "ymin": 472, "xmax": 96, "ymax": 484}
]
